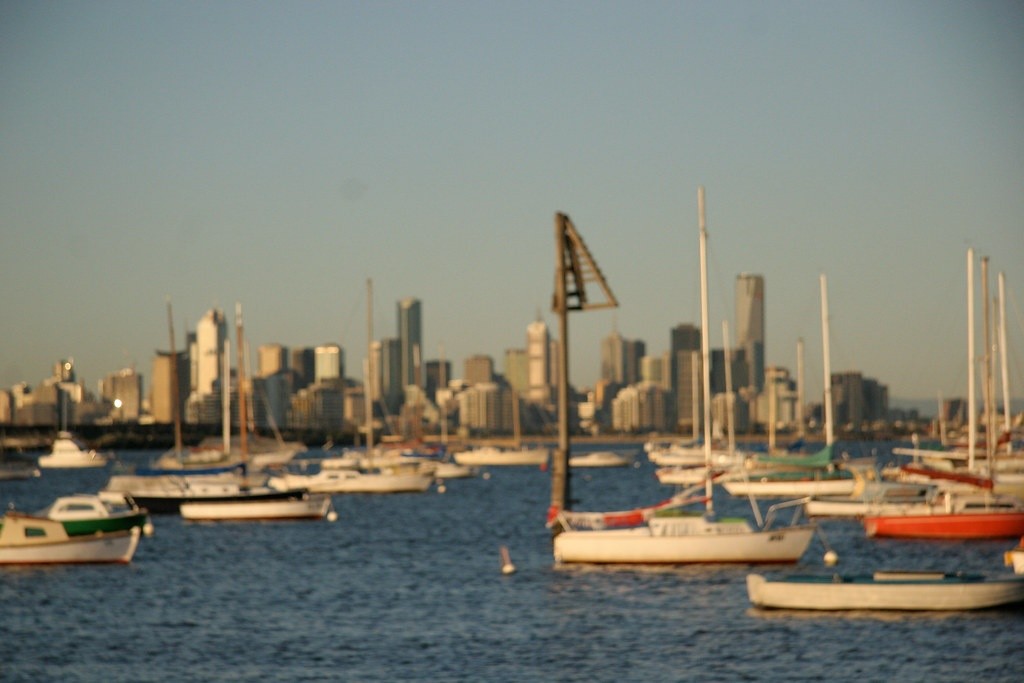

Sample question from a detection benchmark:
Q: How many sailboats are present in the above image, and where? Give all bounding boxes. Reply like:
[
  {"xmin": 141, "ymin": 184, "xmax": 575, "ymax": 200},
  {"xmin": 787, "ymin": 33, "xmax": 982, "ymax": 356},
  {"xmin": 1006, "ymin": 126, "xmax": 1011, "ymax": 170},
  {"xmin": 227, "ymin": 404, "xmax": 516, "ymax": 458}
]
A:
[{"xmin": 640, "ymin": 244, "xmax": 1024, "ymax": 539}]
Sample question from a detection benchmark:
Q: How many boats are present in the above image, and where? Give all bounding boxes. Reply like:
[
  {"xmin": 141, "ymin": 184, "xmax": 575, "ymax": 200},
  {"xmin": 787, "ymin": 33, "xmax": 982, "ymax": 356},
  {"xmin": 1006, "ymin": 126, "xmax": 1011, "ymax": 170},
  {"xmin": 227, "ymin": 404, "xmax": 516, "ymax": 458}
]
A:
[
  {"xmin": 552, "ymin": 186, "xmax": 816, "ymax": 566},
  {"xmin": 0, "ymin": 457, "xmax": 42, "ymax": 480},
  {"xmin": 96, "ymin": 275, "xmax": 552, "ymax": 504},
  {"xmin": 38, "ymin": 439, "xmax": 110, "ymax": 470},
  {"xmin": 745, "ymin": 568, "xmax": 1022, "ymax": 610},
  {"xmin": 27, "ymin": 490, "xmax": 150, "ymax": 537},
  {"xmin": 567, "ymin": 449, "xmax": 627, "ymax": 470},
  {"xmin": 0, "ymin": 511, "xmax": 142, "ymax": 564},
  {"xmin": 178, "ymin": 494, "xmax": 339, "ymax": 521}
]
